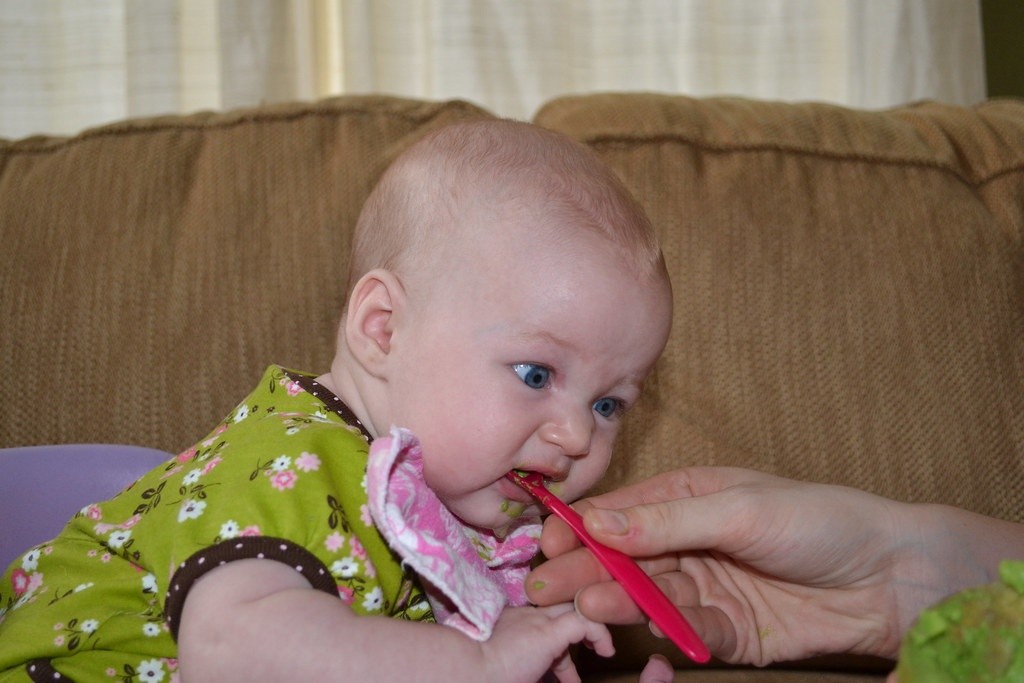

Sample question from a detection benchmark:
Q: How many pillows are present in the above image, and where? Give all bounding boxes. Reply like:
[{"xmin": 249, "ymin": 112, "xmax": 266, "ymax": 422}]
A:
[{"xmin": 2, "ymin": 445, "xmax": 181, "ymax": 578}]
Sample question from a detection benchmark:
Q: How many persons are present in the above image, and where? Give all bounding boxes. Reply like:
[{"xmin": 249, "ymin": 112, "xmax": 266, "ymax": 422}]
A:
[
  {"xmin": 525, "ymin": 467, "xmax": 1024, "ymax": 681},
  {"xmin": 0, "ymin": 121, "xmax": 668, "ymax": 683}
]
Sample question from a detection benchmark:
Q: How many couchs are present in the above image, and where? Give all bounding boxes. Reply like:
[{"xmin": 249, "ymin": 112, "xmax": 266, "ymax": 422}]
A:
[{"xmin": 0, "ymin": 92, "xmax": 1024, "ymax": 682}]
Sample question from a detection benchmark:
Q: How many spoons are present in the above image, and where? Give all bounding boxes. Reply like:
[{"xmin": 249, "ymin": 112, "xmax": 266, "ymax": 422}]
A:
[{"xmin": 505, "ymin": 470, "xmax": 711, "ymax": 665}]
